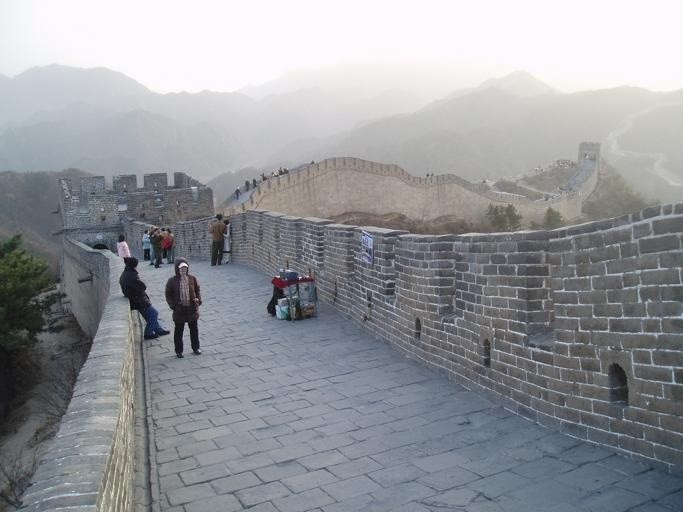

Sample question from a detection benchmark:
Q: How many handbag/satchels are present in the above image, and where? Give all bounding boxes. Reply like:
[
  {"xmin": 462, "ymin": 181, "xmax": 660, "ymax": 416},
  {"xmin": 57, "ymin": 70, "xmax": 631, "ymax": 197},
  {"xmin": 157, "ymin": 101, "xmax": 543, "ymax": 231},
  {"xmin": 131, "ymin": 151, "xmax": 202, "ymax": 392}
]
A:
[{"xmin": 163, "ymin": 234, "xmax": 171, "ymax": 250}]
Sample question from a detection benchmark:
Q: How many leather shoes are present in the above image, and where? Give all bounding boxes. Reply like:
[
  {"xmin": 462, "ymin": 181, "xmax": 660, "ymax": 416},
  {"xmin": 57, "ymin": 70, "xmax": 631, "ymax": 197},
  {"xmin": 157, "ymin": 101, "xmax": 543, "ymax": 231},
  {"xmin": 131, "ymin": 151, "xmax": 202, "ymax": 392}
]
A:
[
  {"xmin": 154, "ymin": 265, "xmax": 160, "ymax": 268},
  {"xmin": 176, "ymin": 352, "xmax": 185, "ymax": 360},
  {"xmin": 159, "ymin": 330, "xmax": 170, "ymax": 337},
  {"xmin": 194, "ymin": 348, "xmax": 203, "ymax": 356},
  {"xmin": 144, "ymin": 333, "xmax": 159, "ymax": 340}
]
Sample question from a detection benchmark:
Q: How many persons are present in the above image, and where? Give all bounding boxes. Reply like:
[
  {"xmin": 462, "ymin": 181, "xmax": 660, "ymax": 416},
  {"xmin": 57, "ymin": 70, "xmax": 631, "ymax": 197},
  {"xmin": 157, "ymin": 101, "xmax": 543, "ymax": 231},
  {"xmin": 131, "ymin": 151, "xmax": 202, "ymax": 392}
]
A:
[
  {"xmin": 166, "ymin": 229, "xmax": 176, "ymax": 264},
  {"xmin": 149, "ymin": 226, "xmax": 155, "ymax": 265},
  {"xmin": 117, "ymin": 236, "xmax": 131, "ymax": 257},
  {"xmin": 165, "ymin": 257, "xmax": 203, "ymax": 358},
  {"xmin": 222, "ymin": 220, "xmax": 231, "ymax": 263},
  {"xmin": 160, "ymin": 228, "xmax": 167, "ymax": 258},
  {"xmin": 142, "ymin": 230, "xmax": 151, "ymax": 260},
  {"xmin": 150, "ymin": 228, "xmax": 164, "ymax": 268},
  {"xmin": 119, "ymin": 258, "xmax": 170, "ymax": 340},
  {"xmin": 208, "ymin": 214, "xmax": 227, "ymax": 268}
]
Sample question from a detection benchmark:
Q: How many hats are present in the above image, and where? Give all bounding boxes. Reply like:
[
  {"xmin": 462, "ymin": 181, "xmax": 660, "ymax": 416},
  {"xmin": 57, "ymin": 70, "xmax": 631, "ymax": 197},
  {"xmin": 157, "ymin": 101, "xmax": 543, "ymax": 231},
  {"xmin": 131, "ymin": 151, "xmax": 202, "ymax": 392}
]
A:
[
  {"xmin": 123, "ymin": 256, "xmax": 139, "ymax": 268},
  {"xmin": 178, "ymin": 262, "xmax": 189, "ymax": 269}
]
